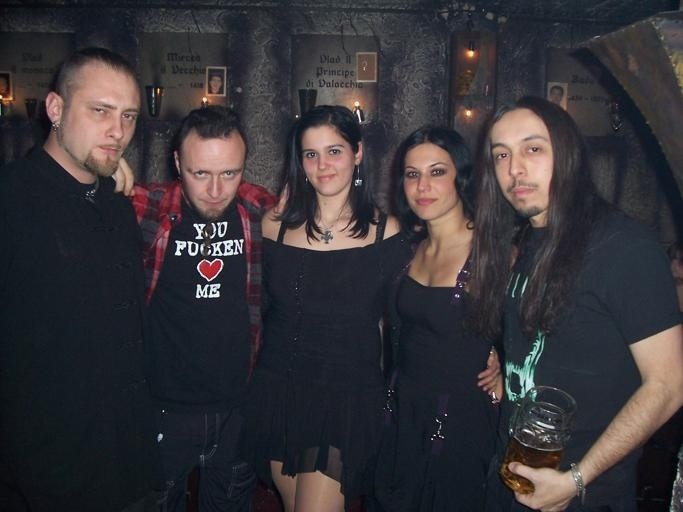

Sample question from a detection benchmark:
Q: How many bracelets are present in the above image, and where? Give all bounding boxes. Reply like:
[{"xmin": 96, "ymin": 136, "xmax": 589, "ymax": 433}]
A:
[{"xmin": 571, "ymin": 460, "xmax": 587, "ymax": 508}]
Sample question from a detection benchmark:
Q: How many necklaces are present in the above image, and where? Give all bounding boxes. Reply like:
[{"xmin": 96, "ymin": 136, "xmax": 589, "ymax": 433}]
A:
[
  {"xmin": 80, "ymin": 176, "xmax": 99, "ymax": 206},
  {"xmin": 192, "ymin": 214, "xmax": 219, "ymax": 260},
  {"xmin": 317, "ymin": 198, "xmax": 349, "ymax": 245}
]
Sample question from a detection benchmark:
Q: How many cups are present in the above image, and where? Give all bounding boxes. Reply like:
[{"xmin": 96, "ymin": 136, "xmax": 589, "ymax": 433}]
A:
[{"xmin": 502, "ymin": 386, "xmax": 578, "ymax": 492}]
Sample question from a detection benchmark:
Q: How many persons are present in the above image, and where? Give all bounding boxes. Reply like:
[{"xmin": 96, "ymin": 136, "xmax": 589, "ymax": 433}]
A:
[
  {"xmin": 466, "ymin": 95, "xmax": 683, "ymax": 512},
  {"xmin": 0, "ymin": 75, "xmax": 9, "ymax": 98},
  {"xmin": 0, "ymin": 48, "xmax": 148, "ymax": 512},
  {"xmin": 109, "ymin": 105, "xmax": 279, "ymax": 512},
  {"xmin": 550, "ymin": 86, "xmax": 564, "ymax": 105},
  {"xmin": 374, "ymin": 123, "xmax": 520, "ymax": 511},
  {"xmin": 260, "ymin": 103, "xmax": 423, "ymax": 511},
  {"xmin": 208, "ymin": 73, "xmax": 224, "ymax": 94}
]
